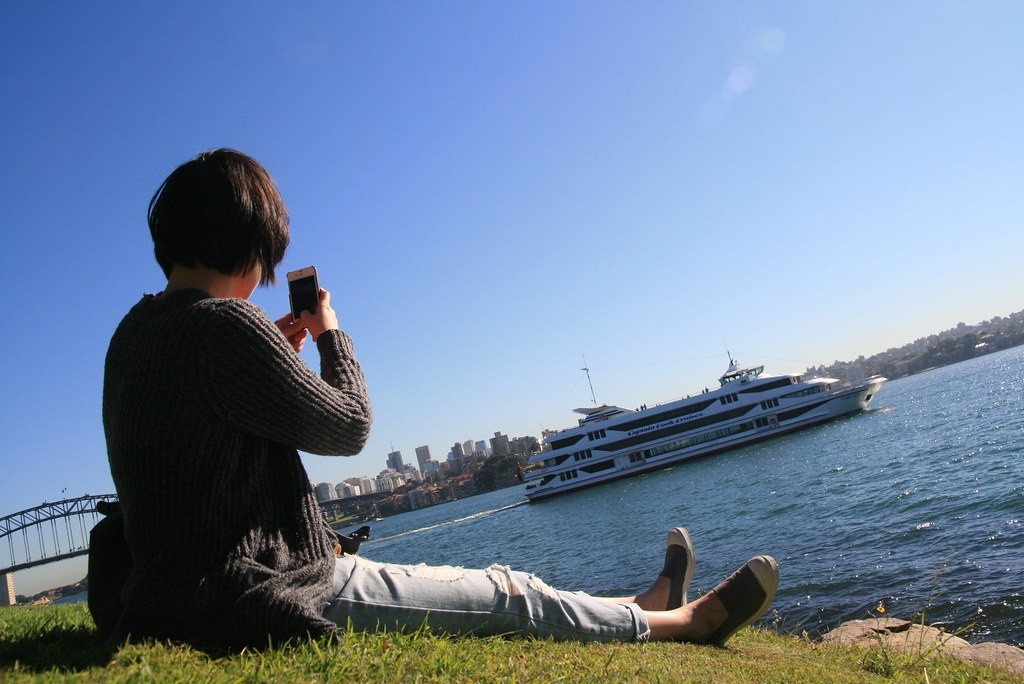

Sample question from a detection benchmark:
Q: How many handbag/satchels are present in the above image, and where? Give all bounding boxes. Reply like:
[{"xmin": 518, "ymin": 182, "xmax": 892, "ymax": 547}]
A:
[{"xmin": 87, "ymin": 502, "xmax": 134, "ymax": 638}]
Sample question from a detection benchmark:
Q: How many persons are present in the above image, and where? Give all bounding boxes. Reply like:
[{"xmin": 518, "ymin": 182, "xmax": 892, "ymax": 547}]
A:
[{"xmin": 102, "ymin": 146, "xmax": 779, "ymax": 650}]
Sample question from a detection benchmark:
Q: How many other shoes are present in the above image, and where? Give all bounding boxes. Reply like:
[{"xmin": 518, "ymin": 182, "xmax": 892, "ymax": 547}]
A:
[
  {"xmin": 658, "ymin": 527, "xmax": 694, "ymax": 612},
  {"xmin": 704, "ymin": 555, "xmax": 780, "ymax": 646}
]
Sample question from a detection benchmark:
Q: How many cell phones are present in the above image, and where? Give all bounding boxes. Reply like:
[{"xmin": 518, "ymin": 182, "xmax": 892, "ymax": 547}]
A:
[{"xmin": 286, "ymin": 266, "xmax": 321, "ymax": 328}]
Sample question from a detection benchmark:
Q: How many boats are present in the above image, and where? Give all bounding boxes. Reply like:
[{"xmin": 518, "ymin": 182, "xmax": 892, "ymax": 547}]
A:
[{"xmin": 521, "ymin": 344, "xmax": 889, "ymax": 502}]
